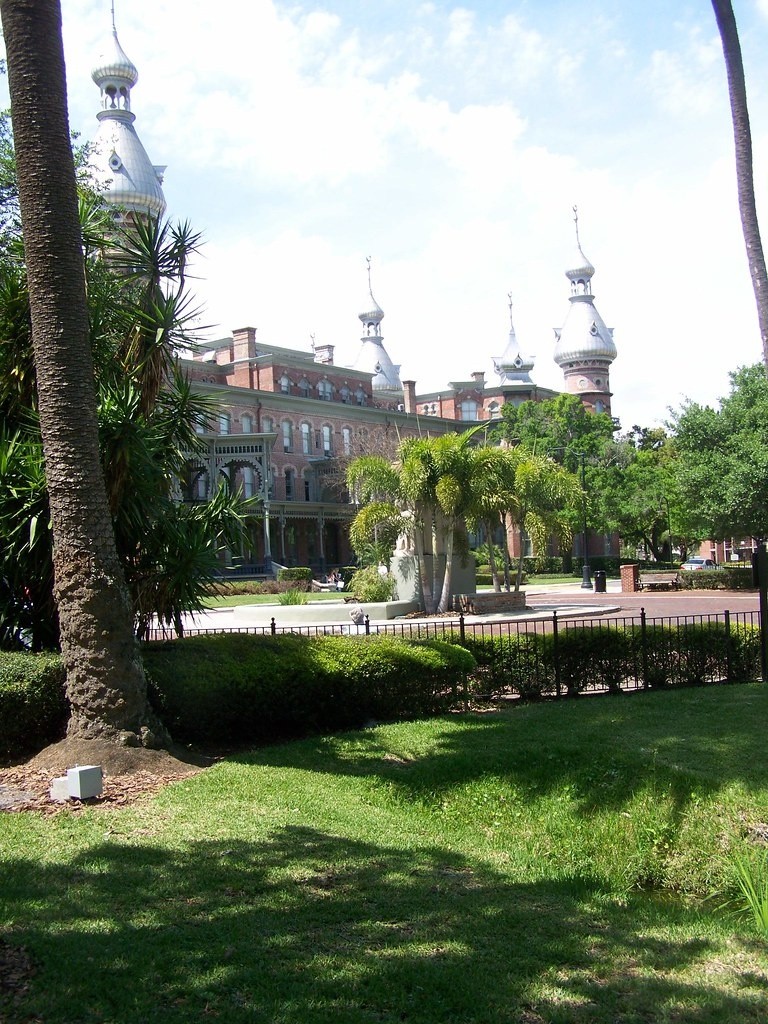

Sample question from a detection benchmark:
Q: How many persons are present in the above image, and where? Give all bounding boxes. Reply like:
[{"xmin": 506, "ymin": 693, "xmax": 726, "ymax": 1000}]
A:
[
  {"xmin": 378, "ymin": 561, "xmax": 388, "ymax": 577},
  {"xmin": 326, "ymin": 568, "xmax": 342, "ymax": 591}
]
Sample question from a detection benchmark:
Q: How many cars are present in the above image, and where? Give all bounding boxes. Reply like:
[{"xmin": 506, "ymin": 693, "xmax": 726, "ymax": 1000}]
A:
[{"xmin": 680, "ymin": 558, "xmax": 726, "ymax": 571}]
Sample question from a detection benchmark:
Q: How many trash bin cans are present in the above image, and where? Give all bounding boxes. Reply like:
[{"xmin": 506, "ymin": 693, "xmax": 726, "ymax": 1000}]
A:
[{"xmin": 594, "ymin": 571, "xmax": 607, "ymax": 592}]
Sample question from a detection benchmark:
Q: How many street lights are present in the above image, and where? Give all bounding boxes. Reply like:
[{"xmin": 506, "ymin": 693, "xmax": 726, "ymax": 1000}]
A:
[{"xmin": 656, "ymin": 496, "xmax": 675, "ymax": 569}]
